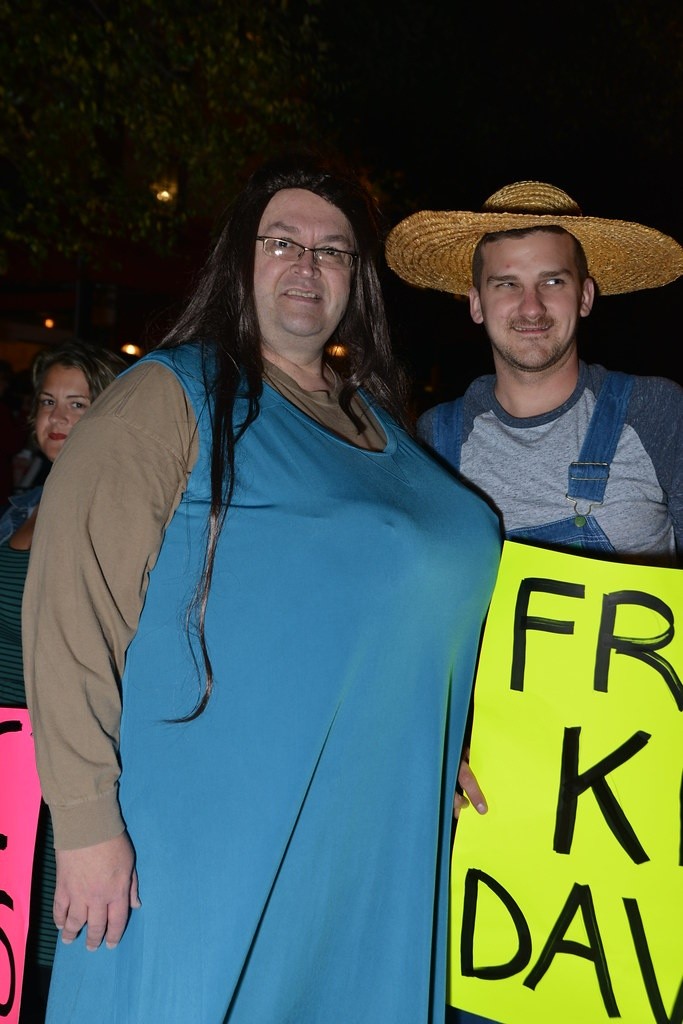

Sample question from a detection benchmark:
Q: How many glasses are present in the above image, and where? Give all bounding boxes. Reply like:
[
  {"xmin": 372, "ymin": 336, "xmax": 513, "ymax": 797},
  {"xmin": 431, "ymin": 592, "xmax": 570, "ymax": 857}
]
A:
[{"xmin": 256, "ymin": 235, "xmax": 360, "ymax": 269}]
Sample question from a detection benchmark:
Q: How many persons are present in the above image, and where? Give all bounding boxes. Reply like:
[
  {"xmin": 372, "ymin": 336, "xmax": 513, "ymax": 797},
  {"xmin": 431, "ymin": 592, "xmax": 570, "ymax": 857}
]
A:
[{"xmin": 1, "ymin": 169, "xmax": 683, "ymax": 1024}]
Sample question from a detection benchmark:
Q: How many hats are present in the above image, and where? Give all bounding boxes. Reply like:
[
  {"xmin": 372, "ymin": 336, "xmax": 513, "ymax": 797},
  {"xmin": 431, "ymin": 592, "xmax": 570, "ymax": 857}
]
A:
[{"xmin": 383, "ymin": 181, "xmax": 683, "ymax": 295}]
